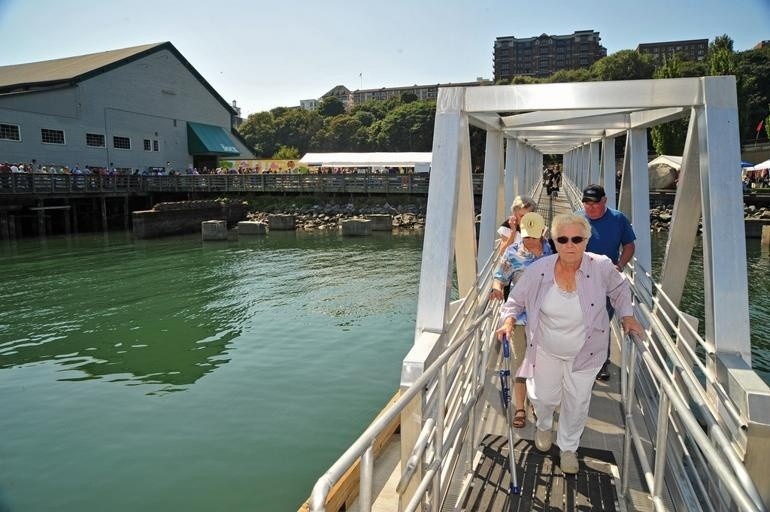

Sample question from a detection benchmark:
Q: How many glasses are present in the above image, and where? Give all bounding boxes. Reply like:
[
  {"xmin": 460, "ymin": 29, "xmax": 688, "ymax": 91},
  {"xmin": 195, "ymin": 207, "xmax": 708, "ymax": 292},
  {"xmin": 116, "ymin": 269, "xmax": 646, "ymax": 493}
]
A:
[{"xmin": 557, "ymin": 236, "xmax": 583, "ymax": 244}]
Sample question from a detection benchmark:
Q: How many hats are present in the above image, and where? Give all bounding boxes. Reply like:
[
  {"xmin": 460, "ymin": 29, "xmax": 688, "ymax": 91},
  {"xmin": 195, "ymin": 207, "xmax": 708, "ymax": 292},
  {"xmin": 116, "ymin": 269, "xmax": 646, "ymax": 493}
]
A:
[
  {"xmin": 520, "ymin": 212, "xmax": 544, "ymax": 239},
  {"xmin": 582, "ymin": 184, "xmax": 605, "ymax": 203}
]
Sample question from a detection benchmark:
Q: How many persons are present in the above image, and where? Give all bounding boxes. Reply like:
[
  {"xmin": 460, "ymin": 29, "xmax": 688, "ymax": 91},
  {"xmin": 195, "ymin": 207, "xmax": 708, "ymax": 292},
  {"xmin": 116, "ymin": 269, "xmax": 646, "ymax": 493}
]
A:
[
  {"xmin": 571, "ymin": 183, "xmax": 636, "ymax": 381},
  {"xmin": 497, "ymin": 195, "xmax": 549, "ymax": 256},
  {"xmin": 616, "ymin": 172, "xmax": 622, "ymax": 190},
  {"xmin": 544, "ymin": 164, "xmax": 561, "ymax": 200},
  {"xmin": 490, "ymin": 211, "xmax": 558, "ymax": 428},
  {"xmin": 748, "ymin": 170, "xmax": 770, "ymax": 189},
  {"xmin": 0, "ymin": 158, "xmax": 414, "ymax": 193},
  {"xmin": 497, "ymin": 212, "xmax": 648, "ymax": 474}
]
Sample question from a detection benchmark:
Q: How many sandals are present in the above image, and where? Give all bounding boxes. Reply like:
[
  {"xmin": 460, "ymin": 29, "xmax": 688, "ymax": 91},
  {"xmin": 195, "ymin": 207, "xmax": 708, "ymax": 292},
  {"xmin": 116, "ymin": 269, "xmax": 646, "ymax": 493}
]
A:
[{"xmin": 514, "ymin": 410, "xmax": 525, "ymax": 428}]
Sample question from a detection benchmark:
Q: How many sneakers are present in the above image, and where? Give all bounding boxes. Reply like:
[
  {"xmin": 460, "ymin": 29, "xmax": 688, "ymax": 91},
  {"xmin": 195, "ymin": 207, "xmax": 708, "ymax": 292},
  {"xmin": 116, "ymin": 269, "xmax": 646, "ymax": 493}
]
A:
[
  {"xmin": 596, "ymin": 362, "xmax": 610, "ymax": 380},
  {"xmin": 534, "ymin": 429, "xmax": 551, "ymax": 451},
  {"xmin": 560, "ymin": 450, "xmax": 579, "ymax": 474}
]
